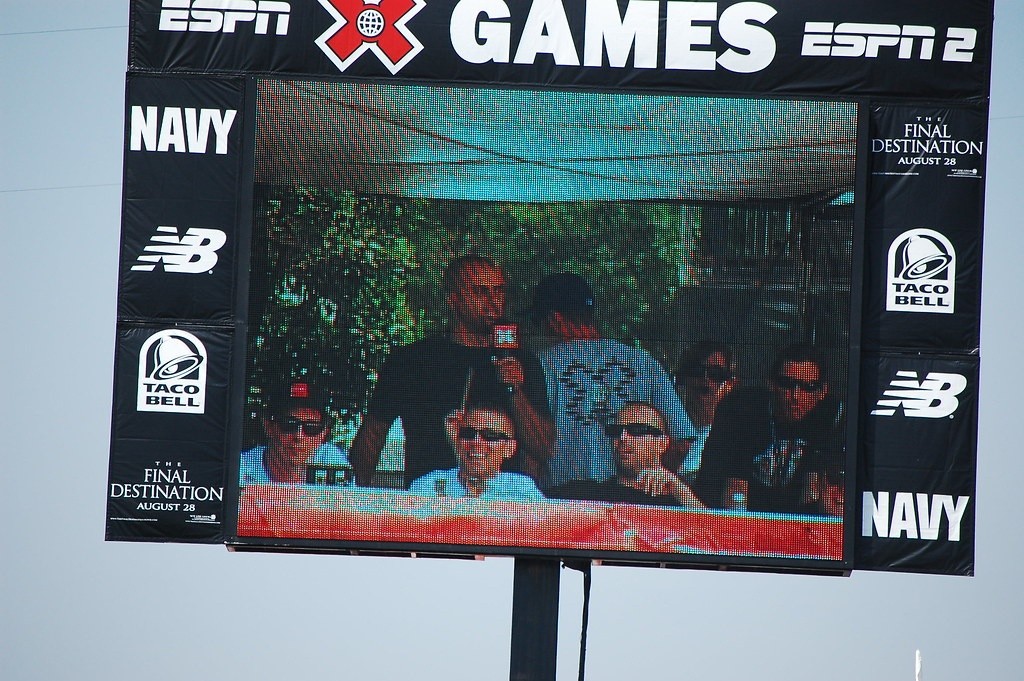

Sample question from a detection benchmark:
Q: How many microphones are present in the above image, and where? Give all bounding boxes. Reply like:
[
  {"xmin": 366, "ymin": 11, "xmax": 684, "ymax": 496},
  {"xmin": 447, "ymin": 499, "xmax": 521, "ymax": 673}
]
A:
[{"xmin": 493, "ymin": 317, "xmax": 518, "ymax": 394}]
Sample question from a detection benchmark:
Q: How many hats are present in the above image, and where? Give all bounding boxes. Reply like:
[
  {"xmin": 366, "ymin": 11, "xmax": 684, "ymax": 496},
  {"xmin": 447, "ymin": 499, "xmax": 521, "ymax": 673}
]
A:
[
  {"xmin": 538, "ymin": 272, "xmax": 595, "ymax": 314},
  {"xmin": 268, "ymin": 380, "xmax": 327, "ymax": 417}
]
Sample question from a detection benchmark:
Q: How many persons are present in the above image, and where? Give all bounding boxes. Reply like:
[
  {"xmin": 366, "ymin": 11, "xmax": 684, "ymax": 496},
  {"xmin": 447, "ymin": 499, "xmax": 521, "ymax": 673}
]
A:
[
  {"xmin": 531, "ymin": 271, "xmax": 700, "ymax": 488},
  {"xmin": 552, "ymin": 403, "xmax": 705, "ymax": 509},
  {"xmin": 238, "ymin": 377, "xmax": 357, "ymax": 486},
  {"xmin": 347, "ymin": 254, "xmax": 557, "ymax": 490},
  {"xmin": 675, "ymin": 338, "xmax": 845, "ymax": 516},
  {"xmin": 407, "ymin": 397, "xmax": 545, "ymax": 499}
]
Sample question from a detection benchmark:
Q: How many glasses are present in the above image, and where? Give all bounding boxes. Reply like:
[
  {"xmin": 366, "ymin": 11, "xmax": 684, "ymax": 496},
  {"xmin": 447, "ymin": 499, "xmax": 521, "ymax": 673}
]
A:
[
  {"xmin": 779, "ymin": 375, "xmax": 827, "ymax": 393},
  {"xmin": 605, "ymin": 423, "xmax": 665, "ymax": 439},
  {"xmin": 280, "ymin": 416, "xmax": 326, "ymax": 436},
  {"xmin": 460, "ymin": 427, "xmax": 511, "ymax": 442},
  {"xmin": 688, "ymin": 364, "xmax": 732, "ymax": 382}
]
